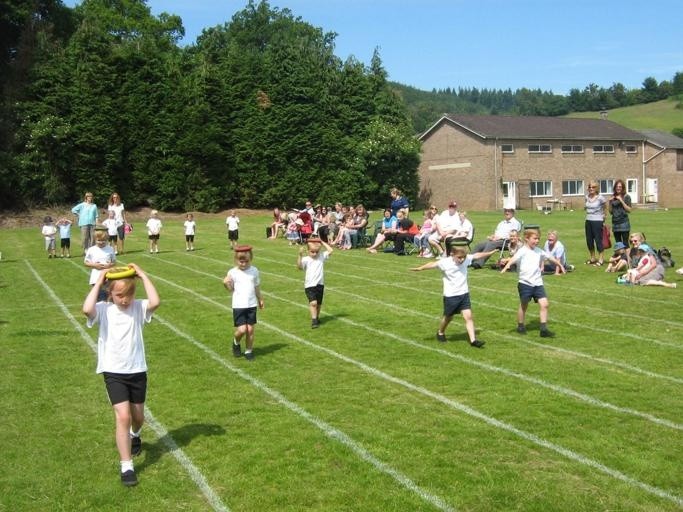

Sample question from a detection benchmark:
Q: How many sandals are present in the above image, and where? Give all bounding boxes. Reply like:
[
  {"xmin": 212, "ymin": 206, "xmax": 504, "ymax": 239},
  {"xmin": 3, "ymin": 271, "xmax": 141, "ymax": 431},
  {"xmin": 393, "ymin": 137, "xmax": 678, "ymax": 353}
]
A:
[
  {"xmin": 583, "ymin": 260, "xmax": 596, "ymax": 265},
  {"xmin": 592, "ymin": 261, "xmax": 603, "ymax": 267}
]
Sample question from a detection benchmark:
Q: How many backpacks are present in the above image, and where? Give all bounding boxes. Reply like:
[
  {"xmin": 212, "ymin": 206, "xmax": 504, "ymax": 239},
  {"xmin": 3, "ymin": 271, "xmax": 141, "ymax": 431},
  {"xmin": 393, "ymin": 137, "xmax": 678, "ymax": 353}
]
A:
[{"xmin": 657, "ymin": 246, "xmax": 676, "ymax": 269}]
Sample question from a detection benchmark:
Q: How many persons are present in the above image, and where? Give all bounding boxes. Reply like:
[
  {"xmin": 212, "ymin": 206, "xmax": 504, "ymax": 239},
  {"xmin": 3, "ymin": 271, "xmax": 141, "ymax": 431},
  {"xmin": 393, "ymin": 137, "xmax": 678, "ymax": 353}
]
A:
[
  {"xmin": 100, "ymin": 209, "xmax": 123, "ymax": 256},
  {"xmin": 41, "ymin": 215, "xmax": 57, "ymax": 259},
  {"xmin": 584, "ymin": 180, "xmax": 677, "ymax": 290},
  {"xmin": 145, "ymin": 208, "xmax": 162, "ymax": 254},
  {"xmin": 181, "ymin": 211, "xmax": 196, "ymax": 251},
  {"xmin": 224, "ymin": 209, "xmax": 240, "ymax": 250},
  {"xmin": 82, "ymin": 262, "xmax": 162, "ymax": 489},
  {"xmin": 413, "ymin": 201, "xmax": 575, "ymax": 276},
  {"xmin": 222, "ymin": 244, "xmax": 266, "ymax": 361},
  {"xmin": 365, "ymin": 187, "xmax": 421, "ymax": 256},
  {"xmin": 267, "ymin": 199, "xmax": 369, "ymax": 249},
  {"xmin": 500, "ymin": 224, "xmax": 566, "ymax": 338},
  {"xmin": 70, "ymin": 192, "xmax": 100, "ymax": 257},
  {"xmin": 105, "ymin": 193, "xmax": 128, "ymax": 255},
  {"xmin": 295, "ymin": 237, "xmax": 334, "ymax": 328},
  {"xmin": 82, "ymin": 225, "xmax": 118, "ymax": 303},
  {"xmin": 53, "ymin": 217, "xmax": 74, "ymax": 258},
  {"xmin": 407, "ymin": 239, "xmax": 500, "ymax": 349}
]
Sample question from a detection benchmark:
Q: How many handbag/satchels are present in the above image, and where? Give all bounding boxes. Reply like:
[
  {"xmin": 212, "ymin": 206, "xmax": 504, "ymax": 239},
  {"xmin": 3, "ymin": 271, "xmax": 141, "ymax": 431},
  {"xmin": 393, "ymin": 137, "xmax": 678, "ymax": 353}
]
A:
[{"xmin": 600, "ymin": 223, "xmax": 611, "ymax": 249}]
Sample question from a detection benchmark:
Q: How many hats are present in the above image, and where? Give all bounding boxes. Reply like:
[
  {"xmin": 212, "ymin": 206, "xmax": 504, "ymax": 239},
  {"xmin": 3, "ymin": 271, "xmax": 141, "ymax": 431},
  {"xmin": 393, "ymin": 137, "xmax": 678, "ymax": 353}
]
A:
[
  {"xmin": 448, "ymin": 201, "xmax": 457, "ymax": 208},
  {"xmin": 613, "ymin": 241, "xmax": 628, "ymax": 252}
]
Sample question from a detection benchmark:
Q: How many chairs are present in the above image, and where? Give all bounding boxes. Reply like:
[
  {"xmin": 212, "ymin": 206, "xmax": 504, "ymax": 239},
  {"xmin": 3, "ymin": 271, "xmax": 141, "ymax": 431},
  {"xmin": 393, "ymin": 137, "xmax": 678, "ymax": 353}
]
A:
[
  {"xmin": 281, "ymin": 220, "xmax": 422, "ymax": 255},
  {"xmin": 496, "ymin": 219, "xmax": 524, "ymax": 266},
  {"xmin": 448, "ymin": 227, "xmax": 476, "ymax": 255}
]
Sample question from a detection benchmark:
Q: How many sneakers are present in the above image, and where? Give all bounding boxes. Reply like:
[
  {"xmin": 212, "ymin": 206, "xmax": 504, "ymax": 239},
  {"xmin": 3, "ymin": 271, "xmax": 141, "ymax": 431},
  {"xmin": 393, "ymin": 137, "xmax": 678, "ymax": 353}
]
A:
[
  {"xmin": 244, "ymin": 351, "xmax": 254, "ymax": 361},
  {"xmin": 46, "ymin": 246, "xmax": 239, "ymax": 260},
  {"xmin": 131, "ymin": 436, "xmax": 141, "ymax": 456},
  {"xmin": 119, "ymin": 470, "xmax": 138, "ymax": 486},
  {"xmin": 539, "ymin": 328, "xmax": 555, "ymax": 338},
  {"xmin": 231, "ymin": 337, "xmax": 240, "ymax": 358},
  {"xmin": 436, "ymin": 330, "xmax": 447, "ymax": 343},
  {"xmin": 517, "ymin": 324, "xmax": 527, "ymax": 336},
  {"xmin": 310, "ymin": 318, "xmax": 320, "ymax": 330},
  {"xmin": 364, "ymin": 243, "xmax": 446, "ymax": 259},
  {"xmin": 469, "ymin": 339, "xmax": 484, "ymax": 348}
]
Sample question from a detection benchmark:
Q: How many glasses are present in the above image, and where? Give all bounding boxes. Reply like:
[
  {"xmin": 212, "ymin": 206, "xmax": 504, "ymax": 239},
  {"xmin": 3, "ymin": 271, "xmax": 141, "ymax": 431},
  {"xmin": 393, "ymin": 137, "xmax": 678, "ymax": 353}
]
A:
[{"xmin": 629, "ymin": 239, "xmax": 638, "ymax": 243}]
